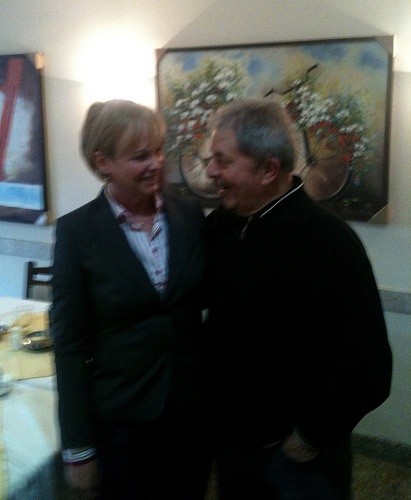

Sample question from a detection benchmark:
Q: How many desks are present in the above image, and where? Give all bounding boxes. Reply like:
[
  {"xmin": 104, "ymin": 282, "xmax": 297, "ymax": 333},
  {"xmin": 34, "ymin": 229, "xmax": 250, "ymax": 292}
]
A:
[{"xmin": 0, "ymin": 297, "xmax": 63, "ymax": 500}]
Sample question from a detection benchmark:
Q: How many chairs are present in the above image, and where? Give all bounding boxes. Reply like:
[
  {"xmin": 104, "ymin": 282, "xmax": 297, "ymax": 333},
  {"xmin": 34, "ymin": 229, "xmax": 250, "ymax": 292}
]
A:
[{"xmin": 27, "ymin": 261, "xmax": 53, "ymax": 302}]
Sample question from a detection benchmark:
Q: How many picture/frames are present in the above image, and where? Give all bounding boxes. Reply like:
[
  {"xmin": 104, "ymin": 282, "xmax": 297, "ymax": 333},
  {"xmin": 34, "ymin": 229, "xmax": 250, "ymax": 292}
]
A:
[
  {"xmin": 156, "ymin": 34, "xmax": 395, "ymax": 226},
  {"xmin": 0, "ymin": 52, "xmax": 49, "ymax": 227}
]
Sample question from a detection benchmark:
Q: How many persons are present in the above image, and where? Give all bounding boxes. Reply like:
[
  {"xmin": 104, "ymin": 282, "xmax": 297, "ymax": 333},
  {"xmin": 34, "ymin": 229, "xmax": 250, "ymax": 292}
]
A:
[
  {"xmin": 201, "ymin": 102, "xmax": 392, "ymax": 499},
  {"xmin": 50, "ymin": 99, "xmax": 211, "ymax": 499}
]
